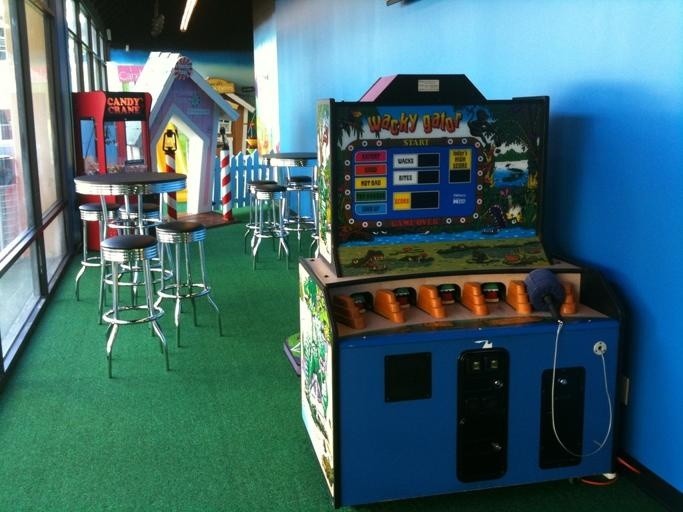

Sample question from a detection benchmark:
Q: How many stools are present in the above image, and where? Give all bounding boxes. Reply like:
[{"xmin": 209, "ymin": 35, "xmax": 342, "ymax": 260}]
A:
[
  {"xmin": 73, "ymin": 166, "xmax": 224, "ymax": 380},
  {"xmin": 243, "ymin": 149, "xmax": 320, "ymax": 268}
]
